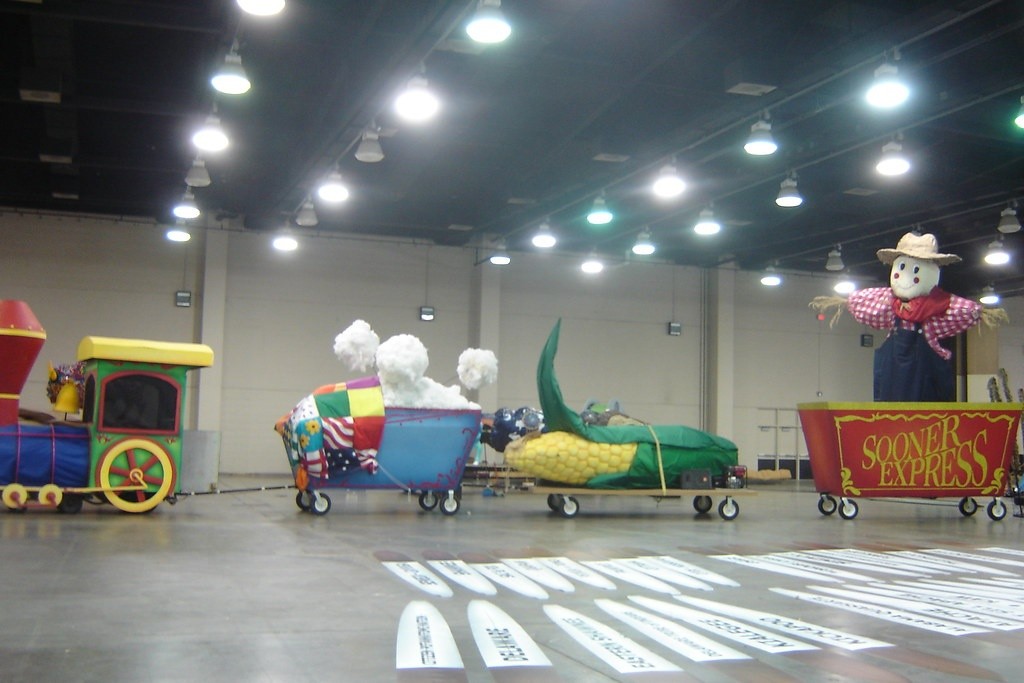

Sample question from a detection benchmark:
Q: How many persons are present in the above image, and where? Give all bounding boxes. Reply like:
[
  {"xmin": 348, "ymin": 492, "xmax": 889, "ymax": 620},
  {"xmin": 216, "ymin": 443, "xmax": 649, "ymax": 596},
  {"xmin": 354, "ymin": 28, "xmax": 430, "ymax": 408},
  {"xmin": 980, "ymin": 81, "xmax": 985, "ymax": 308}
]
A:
[{"xmin": 809, "ymin": 230, "xmax": 1010, "ymax": 402}]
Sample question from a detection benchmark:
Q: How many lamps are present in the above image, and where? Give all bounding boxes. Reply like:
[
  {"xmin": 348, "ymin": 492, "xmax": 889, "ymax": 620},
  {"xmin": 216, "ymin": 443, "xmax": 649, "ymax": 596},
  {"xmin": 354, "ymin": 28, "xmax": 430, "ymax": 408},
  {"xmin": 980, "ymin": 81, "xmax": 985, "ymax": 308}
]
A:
[
  {"xmin": 355, "ymin": 119, "xmax": 385, "ymax": 163},
  {"xmin": 295, "ymin": 195, "xmax": 317, "ymax": 226},
  {"xmin": 586, "ymin": 189, "xmax": 612, "ymax": 225},
  {"xmin": 693, "ymin": 207, "xmax": 721, "ymax": 236},
  {"xmin": 865, "ymin": 62, "xmax": 910, "ymax": 109},
  {"xmin": 489, "ymin": 237, "xmax": 511, "ymax": 266},
  {"xmin": 165, "ymin": 0, "xmax": 285, "ymax": 241},
  {"xmin": 392, "ymin": 61, "xmax": 441, "ymax": 123},
  {"xmin": 775, "ymin": 168, "xmax": 804, "ymax": 208},
  {"xmin": 651, "ymin": 156, "xmax": 686, "ymax": 203},
  {"xmin": 532, "ymin": 217, "xmax": 559, "ymax": 249},
  {"xmin": 318, "ymin": 159, "xmax": 349, "ymax": 203},
  {"xmin": 464, "ymin": 0, "xmax": 511, "ymax": 43},
  {"xmin": 271, "ymin": 220, "xmax": 298, "ymax": 250},
  {"xmin": 632, "ymin": 226, "xmax": 657, "ymax": 255},
  {"xmin": 743, "ymin": 107, "xmax": 777, "ymax": 156}
]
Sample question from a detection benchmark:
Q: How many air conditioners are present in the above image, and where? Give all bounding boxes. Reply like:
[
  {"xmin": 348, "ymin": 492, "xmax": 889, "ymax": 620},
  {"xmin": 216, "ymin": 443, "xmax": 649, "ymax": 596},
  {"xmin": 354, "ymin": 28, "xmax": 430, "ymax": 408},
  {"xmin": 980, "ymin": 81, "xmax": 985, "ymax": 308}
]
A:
[{"xmin": 876, "ymin": 132, "xmax": 910, "ymax": 176}]
